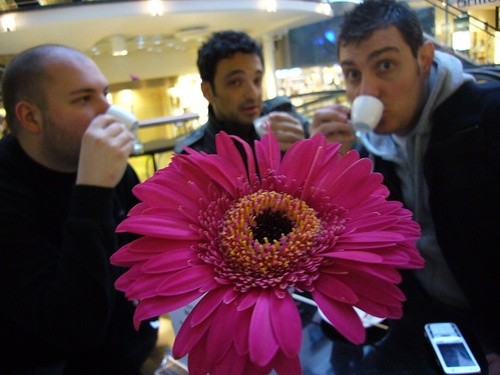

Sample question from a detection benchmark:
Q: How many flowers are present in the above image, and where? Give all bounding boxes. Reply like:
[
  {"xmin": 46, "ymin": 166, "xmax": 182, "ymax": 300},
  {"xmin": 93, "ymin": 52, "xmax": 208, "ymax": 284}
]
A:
[{"xmin": 108, "ymin": 122, "xmax": 425, "ymax": 375}]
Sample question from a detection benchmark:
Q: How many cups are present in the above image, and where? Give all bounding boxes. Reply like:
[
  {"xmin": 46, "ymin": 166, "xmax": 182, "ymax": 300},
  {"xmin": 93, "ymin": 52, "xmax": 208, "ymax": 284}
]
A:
[
  {"xmin": 105, "ymin": 104, "xmax": 139, "ymax": 130},
  {"xmin": 252, "ymin": 116, "xmax": 270, "ymax": 139},
  {"xmin": 347, "ymin": 95, "xmax": 384, "ymax": 132}
]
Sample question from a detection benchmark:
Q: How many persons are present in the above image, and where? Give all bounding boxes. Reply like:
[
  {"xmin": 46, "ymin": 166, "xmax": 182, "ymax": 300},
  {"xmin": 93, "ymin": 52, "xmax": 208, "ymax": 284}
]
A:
[
  {"xmin": 311, "ymin": 0, "xmax": 500, "ymax": 375},
  {"xmin": 0, "ymin": 44, "xmax": 160, "ymax": 375},
  {"xmin": 175, "ymin": 30, "xmax": 313, "ymax": 185}
]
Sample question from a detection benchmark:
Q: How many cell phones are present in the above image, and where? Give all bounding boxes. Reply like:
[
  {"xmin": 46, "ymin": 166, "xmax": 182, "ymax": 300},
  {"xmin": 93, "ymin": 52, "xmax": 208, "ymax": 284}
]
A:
[{"xmin": 424, "ymin": 321, "xmax": 481, "ymax": 375}]
